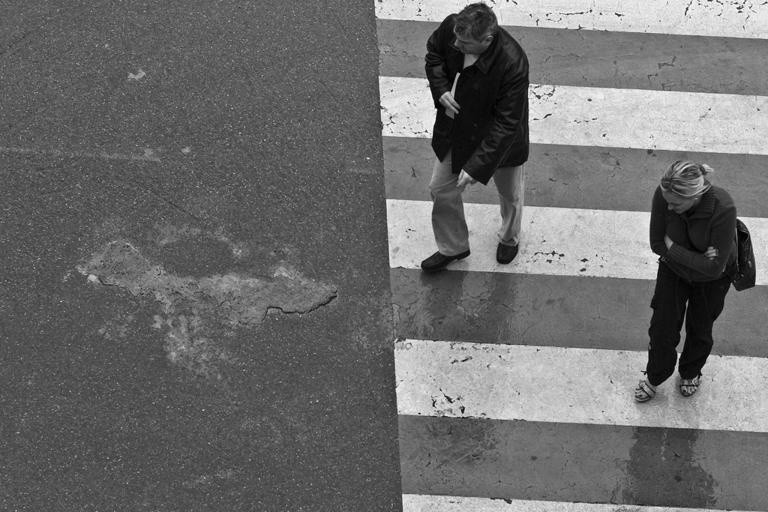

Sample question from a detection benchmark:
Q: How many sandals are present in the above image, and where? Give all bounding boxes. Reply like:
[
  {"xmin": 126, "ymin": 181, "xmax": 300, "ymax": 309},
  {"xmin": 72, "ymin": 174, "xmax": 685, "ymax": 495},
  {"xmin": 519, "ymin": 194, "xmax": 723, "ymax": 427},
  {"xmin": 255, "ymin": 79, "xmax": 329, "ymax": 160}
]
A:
[
  {"xmin": 634, "ymin": 370, "xmax": 657, "ymax": 402},
  {"xmin": 679, "ymin": 372, "xmax": 703, "ymax": 397}
]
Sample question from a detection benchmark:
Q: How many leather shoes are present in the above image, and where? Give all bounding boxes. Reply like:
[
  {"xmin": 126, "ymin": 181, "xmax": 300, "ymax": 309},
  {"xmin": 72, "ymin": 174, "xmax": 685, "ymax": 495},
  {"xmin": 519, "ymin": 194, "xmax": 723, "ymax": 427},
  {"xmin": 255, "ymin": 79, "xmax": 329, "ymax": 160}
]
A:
[
  {"xmin": 420, "ymin": 247, "xmax": 472, "ymax": 274},
  {"xmin": 496, "ymin": 242, "xmax": 518, "ymax": 265}
]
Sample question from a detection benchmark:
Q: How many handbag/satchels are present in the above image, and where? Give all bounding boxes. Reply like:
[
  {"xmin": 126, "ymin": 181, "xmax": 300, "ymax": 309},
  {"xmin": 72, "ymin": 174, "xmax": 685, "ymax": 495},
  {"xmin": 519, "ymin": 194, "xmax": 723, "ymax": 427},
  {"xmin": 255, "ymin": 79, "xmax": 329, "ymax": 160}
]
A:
[{"xmin": 723, "ymin": 217, "xmax": 757, "ymax": 292}]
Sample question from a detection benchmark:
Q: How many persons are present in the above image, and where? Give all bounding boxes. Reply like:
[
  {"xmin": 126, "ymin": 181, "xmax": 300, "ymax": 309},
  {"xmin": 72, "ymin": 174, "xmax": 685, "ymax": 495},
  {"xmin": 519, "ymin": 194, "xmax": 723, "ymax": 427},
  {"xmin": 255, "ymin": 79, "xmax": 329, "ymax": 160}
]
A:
[
  {"xmin": 421, "ymin": 3, "xmax": 530, "ymax": 274},
  {"xmin": 634, "ymin": 161, "xmax": 738, "ymax": 403}
]
